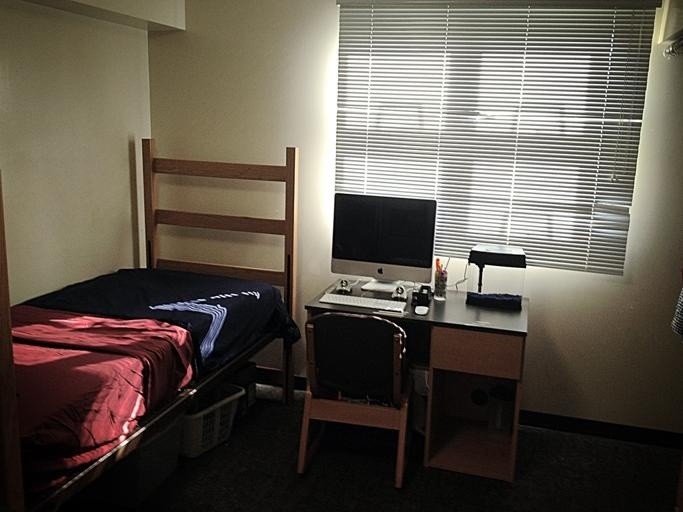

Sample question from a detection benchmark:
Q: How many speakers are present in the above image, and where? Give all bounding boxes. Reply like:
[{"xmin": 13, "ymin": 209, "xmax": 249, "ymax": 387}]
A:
[
  {"xmin": 392, "ymin": 286, "xmax": 408, "ymax": 299},
  {"xmin": 336, "ymin": 279, "xmax": 352, "ymax": 292}
]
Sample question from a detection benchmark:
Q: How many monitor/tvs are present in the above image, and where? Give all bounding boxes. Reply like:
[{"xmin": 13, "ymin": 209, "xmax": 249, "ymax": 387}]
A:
[{"xmin": 329, "ymin": 192, "xmax": 437, "ymax": 292}]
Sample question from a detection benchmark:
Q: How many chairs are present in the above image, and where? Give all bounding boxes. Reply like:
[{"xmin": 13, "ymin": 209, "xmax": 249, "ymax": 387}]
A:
[{"xmin": 297, "ymin": 312, "xmax": 417, "ymax": 489}]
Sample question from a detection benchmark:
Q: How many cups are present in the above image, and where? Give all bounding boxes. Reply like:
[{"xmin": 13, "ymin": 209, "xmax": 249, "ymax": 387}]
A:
[{"xmin": 434, "ymin": 268, "xmax": 447, "ymax": 301}]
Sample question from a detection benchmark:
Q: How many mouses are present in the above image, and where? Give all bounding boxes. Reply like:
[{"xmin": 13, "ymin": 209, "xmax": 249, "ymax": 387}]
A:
[{"xmin": 415, "ymin": 306, "xmax": 429, "ymax": 316}]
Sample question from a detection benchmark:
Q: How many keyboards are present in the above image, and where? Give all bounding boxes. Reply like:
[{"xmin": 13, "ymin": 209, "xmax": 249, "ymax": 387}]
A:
[{"xmin": 319, "ymin": 293, "xmax": 407, "ymax": 313}]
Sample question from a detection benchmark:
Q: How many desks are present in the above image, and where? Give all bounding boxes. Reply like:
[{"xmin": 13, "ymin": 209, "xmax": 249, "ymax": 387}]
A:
[{"xmin": 305, "ymin": 278, "xmax": 529, "ymax": 483}]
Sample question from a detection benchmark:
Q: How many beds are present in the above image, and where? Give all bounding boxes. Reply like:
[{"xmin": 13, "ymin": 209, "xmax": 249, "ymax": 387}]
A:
[{"xmin": 1, "ymin": 138, "xmax": 298, "ymax": 512}]
